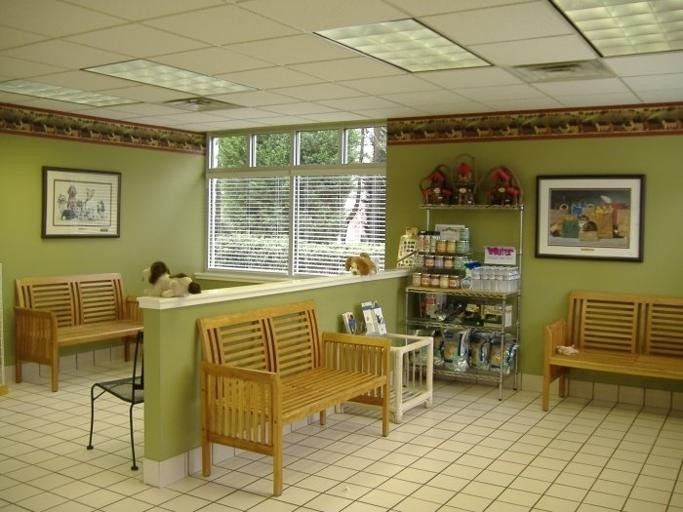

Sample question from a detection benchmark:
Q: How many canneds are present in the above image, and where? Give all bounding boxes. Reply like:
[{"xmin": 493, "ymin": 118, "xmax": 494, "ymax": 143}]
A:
[{"xmin": 411, "ymin": 239, "xmax": 470, "ymax": 288}]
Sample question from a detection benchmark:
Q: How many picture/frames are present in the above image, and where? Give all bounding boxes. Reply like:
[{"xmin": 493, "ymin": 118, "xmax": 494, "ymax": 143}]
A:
[
  {"xmin": 43, "ymin": 168, "xmax": 122, "ymax": 237},
  {"xmin": 536, "ymin": 175, "xmax": 646, "ymax": 262}
]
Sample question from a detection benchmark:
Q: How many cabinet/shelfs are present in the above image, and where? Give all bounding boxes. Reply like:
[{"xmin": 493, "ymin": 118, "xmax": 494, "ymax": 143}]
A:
[{"xmin": 401, "ymin": 204, "xmax": 525, "ymax": 400}]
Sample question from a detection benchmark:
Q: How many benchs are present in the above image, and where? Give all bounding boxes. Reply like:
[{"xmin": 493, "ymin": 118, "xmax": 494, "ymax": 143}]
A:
[
  {"xmin": 543, "ymin": 291, "xmax": 682, "ymax": 413},
  {"xmin": 12, "ymin": 272, "xmax": 144, "ymax": 392},
  {"xmin": 197, "ymin": 300, "xmax": 391, "ymax": 498}
]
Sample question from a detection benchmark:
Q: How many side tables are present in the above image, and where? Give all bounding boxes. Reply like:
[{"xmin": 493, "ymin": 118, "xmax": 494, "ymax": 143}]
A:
[{"xmin": 334, "ymin": 333, "xmax": 434, "ymax": 424}]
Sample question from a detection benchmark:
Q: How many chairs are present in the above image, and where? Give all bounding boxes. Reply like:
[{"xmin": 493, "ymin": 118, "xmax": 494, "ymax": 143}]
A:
[{"xmin": 87, "ymin": 330, "xmax": 145, "ymax": 470}]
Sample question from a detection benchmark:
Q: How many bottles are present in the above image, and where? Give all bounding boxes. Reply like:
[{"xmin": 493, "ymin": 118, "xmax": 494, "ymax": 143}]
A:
[
  {"xmin": 470, "ymin": 266, "xmax": 520, "ymax": 293},
  {"xmin": 412, "ymin": 229, "xmax": 480, "ymax": 290}
]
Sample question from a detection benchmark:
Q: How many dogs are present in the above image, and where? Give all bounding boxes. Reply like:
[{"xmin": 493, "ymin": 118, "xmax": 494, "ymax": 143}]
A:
[{"xmin": 57, "ymin": 185, "xmax": 106, "ymax": 221}]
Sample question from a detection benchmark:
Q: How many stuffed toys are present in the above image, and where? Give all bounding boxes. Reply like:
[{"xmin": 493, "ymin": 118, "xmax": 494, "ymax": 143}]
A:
[{"xmin": 144, "ymin": 262, "xmax": 201, "ymax": 298}]
